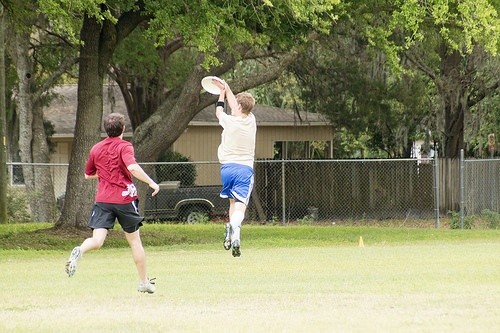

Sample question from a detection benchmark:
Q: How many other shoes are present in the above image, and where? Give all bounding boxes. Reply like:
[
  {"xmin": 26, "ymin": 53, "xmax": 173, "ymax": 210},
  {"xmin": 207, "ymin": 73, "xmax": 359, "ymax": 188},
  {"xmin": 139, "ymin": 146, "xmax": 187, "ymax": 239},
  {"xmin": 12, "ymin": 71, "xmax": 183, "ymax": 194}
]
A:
[
  {"xmin": 65, "ymin": 247, "xmax": 81, "ymax": 277},
  {"xmin": 138, "ymin": 278, "xmax": 155, "ymax": 293},
  {"xmin": 232, "ymin": 239, "xmax": 241, "ymax": 257},
  {"xmin": 223, "ymin": 223, "xmax": 231, "ymax": 250}
]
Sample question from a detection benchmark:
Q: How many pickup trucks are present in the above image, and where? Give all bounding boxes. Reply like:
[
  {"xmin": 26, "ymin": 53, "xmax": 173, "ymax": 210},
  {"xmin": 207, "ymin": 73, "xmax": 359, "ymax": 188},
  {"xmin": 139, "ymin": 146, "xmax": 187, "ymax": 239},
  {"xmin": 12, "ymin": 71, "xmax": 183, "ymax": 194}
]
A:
[{"xmin": 55, "ymin": 165, "xmax": 230, "ymax": 224}]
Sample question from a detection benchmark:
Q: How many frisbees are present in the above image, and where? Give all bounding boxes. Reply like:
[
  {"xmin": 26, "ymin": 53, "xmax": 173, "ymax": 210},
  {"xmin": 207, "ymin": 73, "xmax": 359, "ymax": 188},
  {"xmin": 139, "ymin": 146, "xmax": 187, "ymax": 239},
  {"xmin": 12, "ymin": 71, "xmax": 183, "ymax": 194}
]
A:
[{"xmin": 201, "ymin": 76, "xmax": 227, "ymax": 94}]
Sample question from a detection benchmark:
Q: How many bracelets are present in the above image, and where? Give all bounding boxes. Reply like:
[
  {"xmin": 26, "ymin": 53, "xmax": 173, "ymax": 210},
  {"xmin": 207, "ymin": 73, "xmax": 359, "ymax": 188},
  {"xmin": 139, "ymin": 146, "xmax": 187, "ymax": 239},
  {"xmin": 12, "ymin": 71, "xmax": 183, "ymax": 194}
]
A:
[{"xmin": 217, "ymin": 102, "xmax": 224, "ymax": 108}]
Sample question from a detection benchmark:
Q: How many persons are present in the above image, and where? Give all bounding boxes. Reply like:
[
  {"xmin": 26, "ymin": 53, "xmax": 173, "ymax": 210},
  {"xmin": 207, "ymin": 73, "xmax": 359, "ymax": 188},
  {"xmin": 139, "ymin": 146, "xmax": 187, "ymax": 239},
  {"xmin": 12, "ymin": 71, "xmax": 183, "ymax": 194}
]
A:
[
  {"xmin": 212, "ymin": 78, "xmax": 257, "ymax": 258},
  {"xmin": 64, "ymin": 113, "xmax": 160, "ymax": 293}
]
